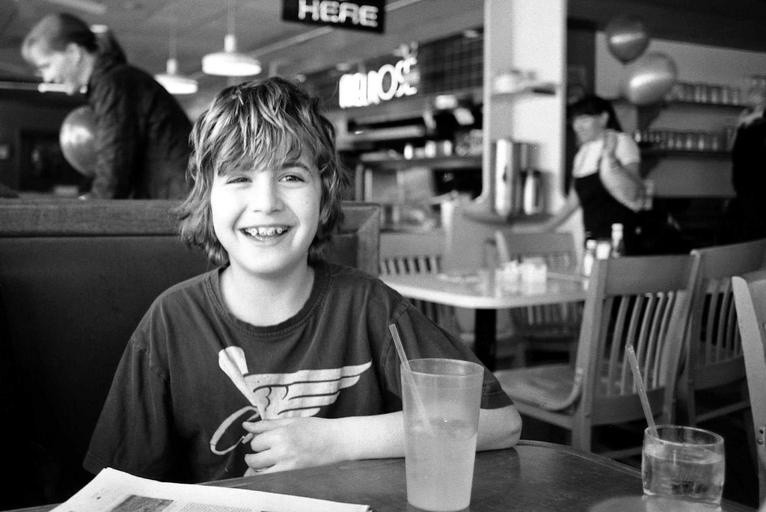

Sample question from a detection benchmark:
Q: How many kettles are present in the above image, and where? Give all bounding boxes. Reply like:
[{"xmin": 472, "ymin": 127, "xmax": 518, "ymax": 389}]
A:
[{"xmin": 522, "ymin": 170, "xmax": 547, "ymax": 221}]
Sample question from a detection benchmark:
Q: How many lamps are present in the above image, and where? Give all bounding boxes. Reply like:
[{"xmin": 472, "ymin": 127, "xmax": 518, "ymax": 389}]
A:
[{"xmin": 154, "ymin": 3, "xmax": 260, "ymax": 94}]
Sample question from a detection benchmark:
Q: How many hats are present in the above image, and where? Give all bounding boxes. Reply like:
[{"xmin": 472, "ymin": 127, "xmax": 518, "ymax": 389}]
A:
[{"xmin": 571, "ymin": 94, "xmax": 606, "ymax": 116}]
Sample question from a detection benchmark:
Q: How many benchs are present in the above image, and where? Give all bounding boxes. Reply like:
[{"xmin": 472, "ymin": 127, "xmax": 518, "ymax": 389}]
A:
[{"xmin": 1, "ymin": 201, "xmax": 384, "ymax": 510}]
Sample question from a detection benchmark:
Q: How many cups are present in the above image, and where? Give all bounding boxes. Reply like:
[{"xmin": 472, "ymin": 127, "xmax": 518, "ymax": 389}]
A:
[
  {"xmin": 400, "ymin": 357, "xmax": 484, "ymax": 509},
  {"xmin": 673, "ymin": 82, "xmax": 740, "ymax": 104},
  {"xmin": 641, "ymin": 424, "xmax": 725, "ymax": 509},
  {"xmin": 655, "ymin": 129, "xmax": 724, "ymax": 149}
]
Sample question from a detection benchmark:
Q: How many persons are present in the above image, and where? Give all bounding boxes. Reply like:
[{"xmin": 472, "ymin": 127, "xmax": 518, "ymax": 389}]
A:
[
  {"xmin": 83, "ymin": 77, "xmax": 523, "ymax": 483},
  {"xmin": 533, "ymin": 93, "xmax": 681, "ymax": 256},
  {"xmin": 727, "ymin": 75, "xmax": 766, "ymax": 244},
  {"xmin": 21, "ymin": 12, "xmax": 194, "ymax": 200}
]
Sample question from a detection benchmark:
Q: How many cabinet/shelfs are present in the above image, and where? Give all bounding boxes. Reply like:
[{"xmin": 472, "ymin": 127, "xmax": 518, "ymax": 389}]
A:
[{"xmin": 632, "ymin": 101, "xmax": 753, "ymax": 160}]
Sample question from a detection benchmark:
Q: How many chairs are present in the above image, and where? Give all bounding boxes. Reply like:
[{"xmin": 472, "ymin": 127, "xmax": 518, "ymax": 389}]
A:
[
  {"xmin": 497, "ymin": 250, "xmax": 703, "ymax": 461},
  {"xmin": 607, "ymin": 241, "xmax": 764, "ymax": 452},
  {"xmin": 733, "ymin": 273, "xmax": 766, "ymax": 493},
  {"xmin": 493, "ymin": 228, "xmax": 590, "ymax": 369},
  {"xmin": 372, "ymin": 231, "xmax": 470, "ymax": 352}
]
